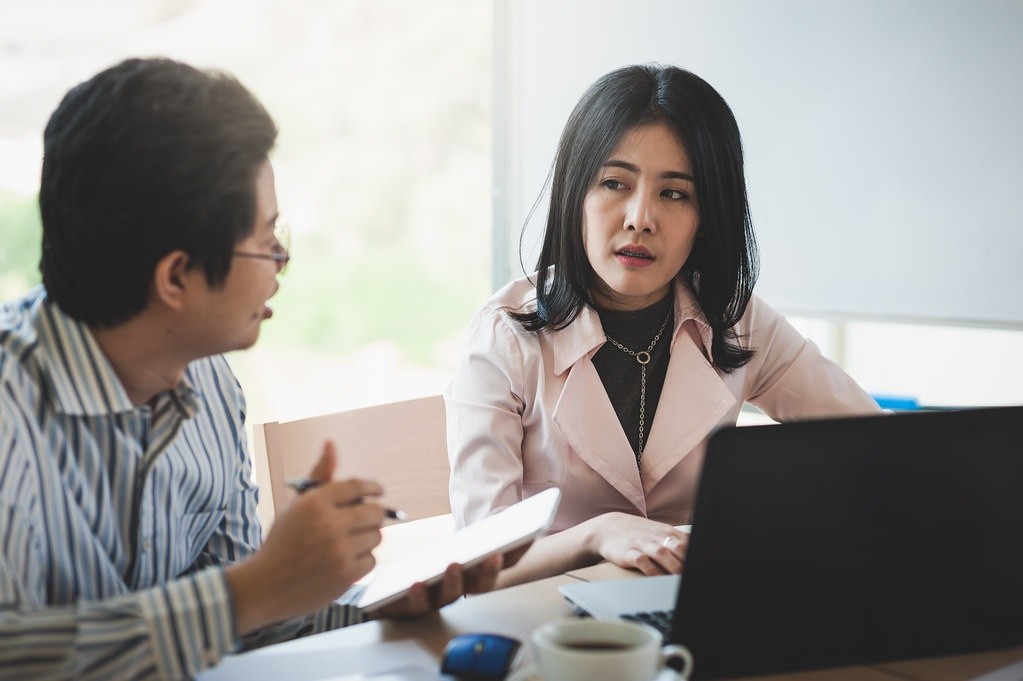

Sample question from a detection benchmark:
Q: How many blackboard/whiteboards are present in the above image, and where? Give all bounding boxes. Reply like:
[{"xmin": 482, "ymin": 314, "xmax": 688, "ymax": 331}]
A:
[{"xmin": 487, "ymin": 0, "xmax": 1023, "ymax": 332}]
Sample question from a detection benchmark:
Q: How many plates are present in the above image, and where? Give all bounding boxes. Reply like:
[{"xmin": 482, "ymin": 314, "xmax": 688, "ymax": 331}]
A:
[{"xmin": 506, "ymin": 661, "xmax": 689, "ymax": 681}]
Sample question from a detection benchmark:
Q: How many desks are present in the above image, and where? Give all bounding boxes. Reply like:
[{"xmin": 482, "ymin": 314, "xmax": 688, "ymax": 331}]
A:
[{"xmin": 194, "ymin": 559, "xmax": 1023, "ymax": 681}]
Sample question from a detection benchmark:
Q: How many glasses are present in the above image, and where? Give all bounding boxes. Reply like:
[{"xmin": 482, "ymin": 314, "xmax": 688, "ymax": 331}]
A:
[{"xmin": 233, "ymin": 225, "xmax": 290, "ymax": 275}]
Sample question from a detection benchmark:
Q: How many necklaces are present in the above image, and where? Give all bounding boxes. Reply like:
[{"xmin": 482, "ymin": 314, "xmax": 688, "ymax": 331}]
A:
[{"xmin": 604, "ymin": 302, "xmax": 674, "ymax": 469}]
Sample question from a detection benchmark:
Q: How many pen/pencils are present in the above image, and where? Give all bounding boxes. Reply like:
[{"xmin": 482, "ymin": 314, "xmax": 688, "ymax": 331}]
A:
[{"xmin": 281, "ymin": 479, "xmax": 408, "ymax": 525}]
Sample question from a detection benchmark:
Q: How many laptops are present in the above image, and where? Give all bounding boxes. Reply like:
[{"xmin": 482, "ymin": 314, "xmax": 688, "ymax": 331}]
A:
[{"xmin": 560, "ymin": 407, "xmax": 1023, "ymax": 681}]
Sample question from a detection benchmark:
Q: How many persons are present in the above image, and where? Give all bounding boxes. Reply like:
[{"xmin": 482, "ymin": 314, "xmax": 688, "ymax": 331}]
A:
[
  {"xmin": 441, "ymin": 66, "xmax": 888, "ymax": 595},
  {"xmin": 0, "ymin": 59, "xmax": 537, "ymax": 681}
]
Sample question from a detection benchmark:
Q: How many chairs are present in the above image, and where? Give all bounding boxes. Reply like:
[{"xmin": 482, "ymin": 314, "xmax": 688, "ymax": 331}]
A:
[{"xmin": 252, "ymin": 394, "xmax": 451, "ymax": 539}]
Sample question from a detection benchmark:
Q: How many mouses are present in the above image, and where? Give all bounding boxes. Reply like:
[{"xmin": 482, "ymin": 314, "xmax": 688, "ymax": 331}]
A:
[{"xmin": 437, "ymin": 634, "xmax": 522, "ymax": 681}]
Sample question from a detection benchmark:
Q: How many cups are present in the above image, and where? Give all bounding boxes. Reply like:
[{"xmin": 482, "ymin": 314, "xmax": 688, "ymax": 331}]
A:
[{"xmin": 533, "ymin": 619, "xmax": 693, "ymax": 681}]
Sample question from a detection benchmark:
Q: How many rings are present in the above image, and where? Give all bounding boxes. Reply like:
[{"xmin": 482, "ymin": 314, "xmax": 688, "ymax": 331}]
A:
[{"xmin": 663, "ymin": 535, "xmax": 679, "ymax": 548}]
